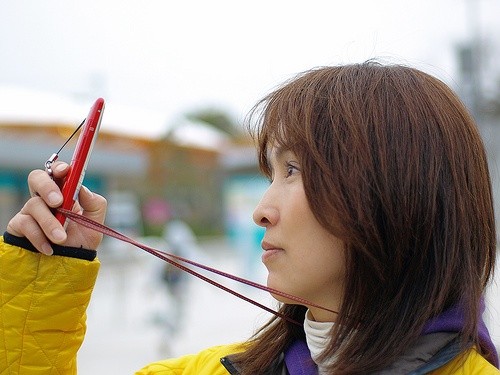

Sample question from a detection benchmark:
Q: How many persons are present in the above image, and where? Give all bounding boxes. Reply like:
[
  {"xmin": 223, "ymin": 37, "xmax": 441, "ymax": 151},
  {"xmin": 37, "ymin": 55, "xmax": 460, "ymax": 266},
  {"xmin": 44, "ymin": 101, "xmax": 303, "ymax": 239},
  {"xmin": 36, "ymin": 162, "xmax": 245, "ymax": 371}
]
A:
[{"xmin": 1, "ymin": 62, "xmax": 499, "ymax": 375}]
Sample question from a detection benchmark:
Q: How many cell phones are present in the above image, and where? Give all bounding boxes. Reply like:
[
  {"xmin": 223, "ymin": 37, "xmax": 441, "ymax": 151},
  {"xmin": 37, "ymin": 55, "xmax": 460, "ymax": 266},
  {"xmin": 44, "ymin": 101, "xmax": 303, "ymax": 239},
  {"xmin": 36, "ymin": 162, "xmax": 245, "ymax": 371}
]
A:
[{"xmin": 52, "ymin": 98, "xmax": 105, "ymax": 231}]
